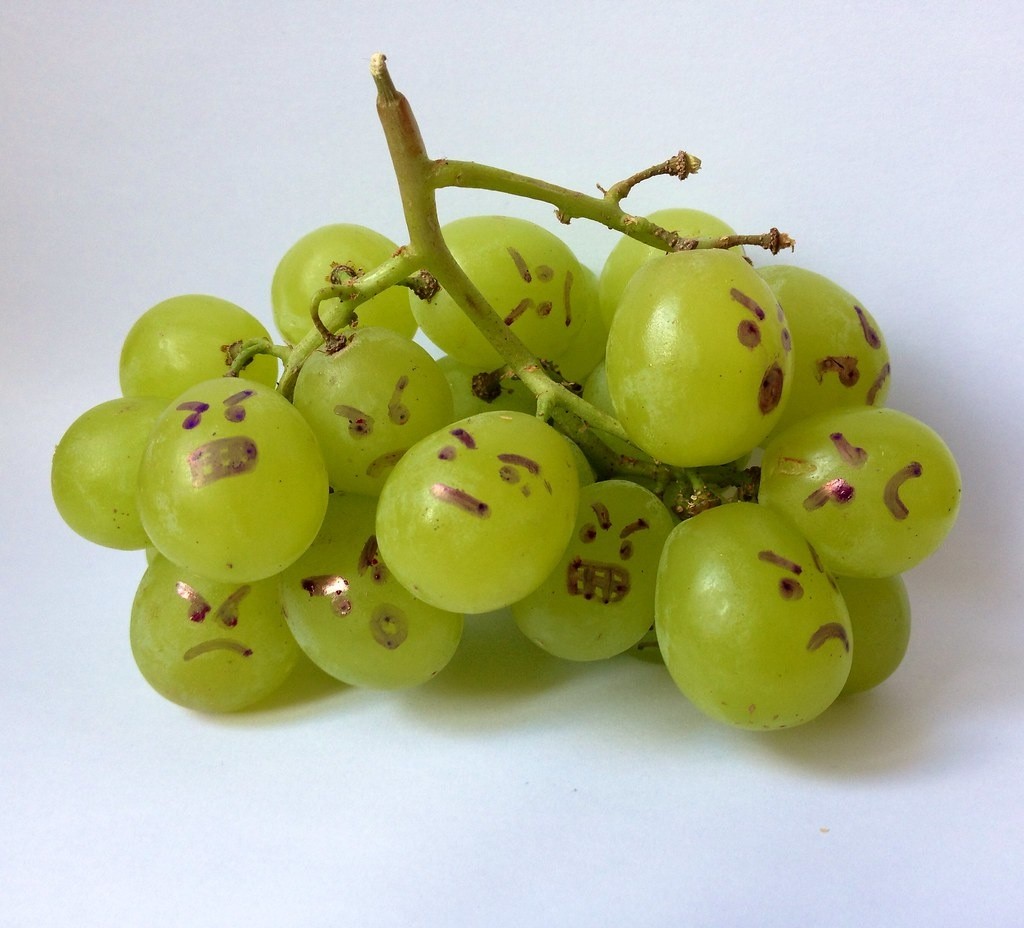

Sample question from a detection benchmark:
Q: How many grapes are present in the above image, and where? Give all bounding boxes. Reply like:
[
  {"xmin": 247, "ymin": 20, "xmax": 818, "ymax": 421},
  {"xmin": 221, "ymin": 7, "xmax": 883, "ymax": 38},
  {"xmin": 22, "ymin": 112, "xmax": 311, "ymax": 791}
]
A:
[{"xmin": 51, "ymin": 210, "xmax": 961, "ymax": 730}]
